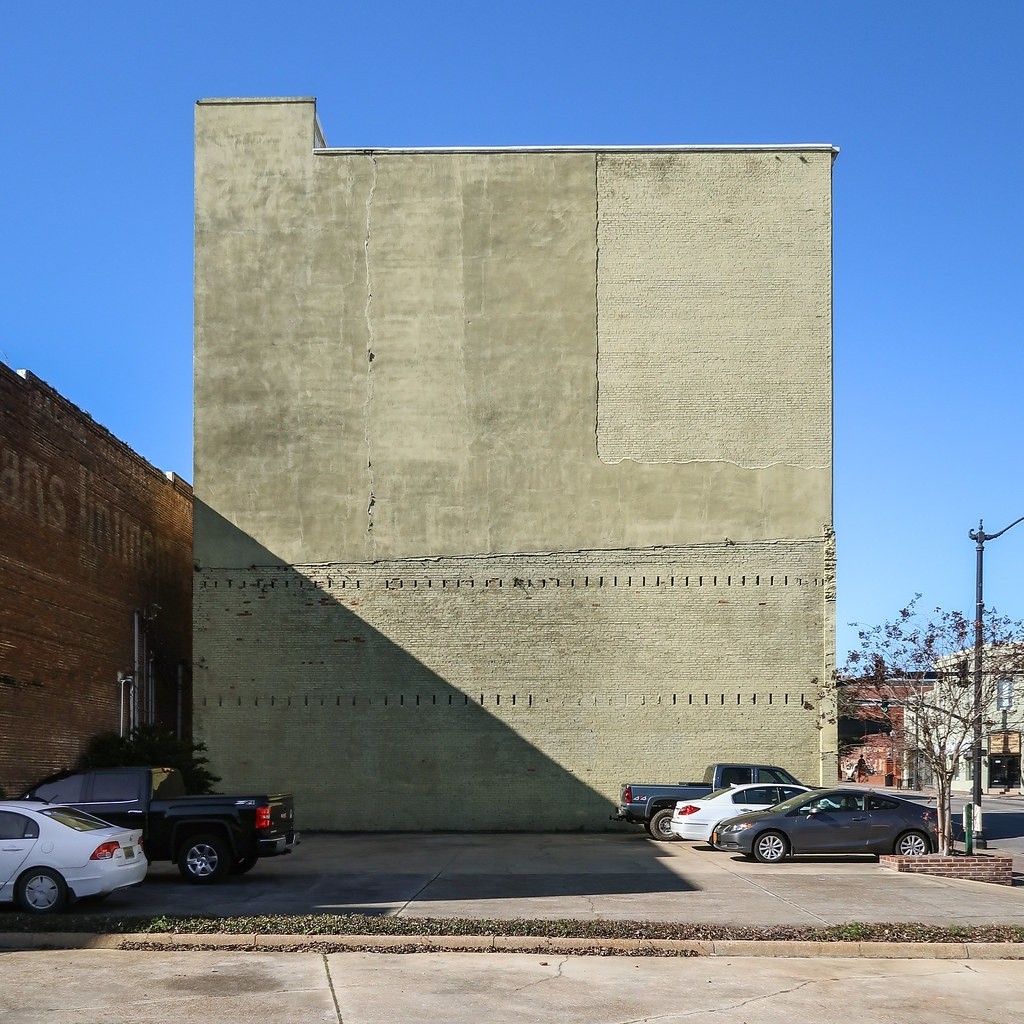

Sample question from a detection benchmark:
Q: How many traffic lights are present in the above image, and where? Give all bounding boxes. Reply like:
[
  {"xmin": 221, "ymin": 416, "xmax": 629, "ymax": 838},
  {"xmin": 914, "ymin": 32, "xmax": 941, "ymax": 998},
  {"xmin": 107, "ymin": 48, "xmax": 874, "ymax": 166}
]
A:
[{"xmin": 882, "ymin": 700, "xmax": 889, "ymax": 714}]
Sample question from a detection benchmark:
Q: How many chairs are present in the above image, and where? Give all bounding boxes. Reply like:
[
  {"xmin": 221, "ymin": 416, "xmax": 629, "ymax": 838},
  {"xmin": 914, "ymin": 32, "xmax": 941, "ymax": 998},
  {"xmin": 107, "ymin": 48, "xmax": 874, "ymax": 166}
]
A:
[{"xmin": 840, "ymin": 798, "xmax": 857, "ymax": 810}]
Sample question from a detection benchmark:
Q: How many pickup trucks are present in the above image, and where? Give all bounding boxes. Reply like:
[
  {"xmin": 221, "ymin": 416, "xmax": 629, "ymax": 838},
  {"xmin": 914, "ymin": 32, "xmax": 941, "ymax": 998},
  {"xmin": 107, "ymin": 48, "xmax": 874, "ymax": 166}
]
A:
[
  {"xmin": 607, "ymin": 762, "xmax": 830, "ymax": 841},
  {"xmin": 18, "ymin": 766, "xmax": 301, "ymax": 883}
]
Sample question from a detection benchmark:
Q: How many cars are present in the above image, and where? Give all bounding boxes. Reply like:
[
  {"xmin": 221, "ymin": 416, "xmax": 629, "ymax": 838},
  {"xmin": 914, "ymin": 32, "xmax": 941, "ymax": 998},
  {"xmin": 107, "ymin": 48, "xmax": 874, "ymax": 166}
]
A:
[
  {"xmin": 672, "ymin": 781, "xmax": 815, "ymax": 849},
  {"xmin": 711, "ymin": 788, "xmax": 955, "ymax": 863},
  {"xmin": 1, "ymin": 799, "xmax": 150, "ymax": 912}
]
